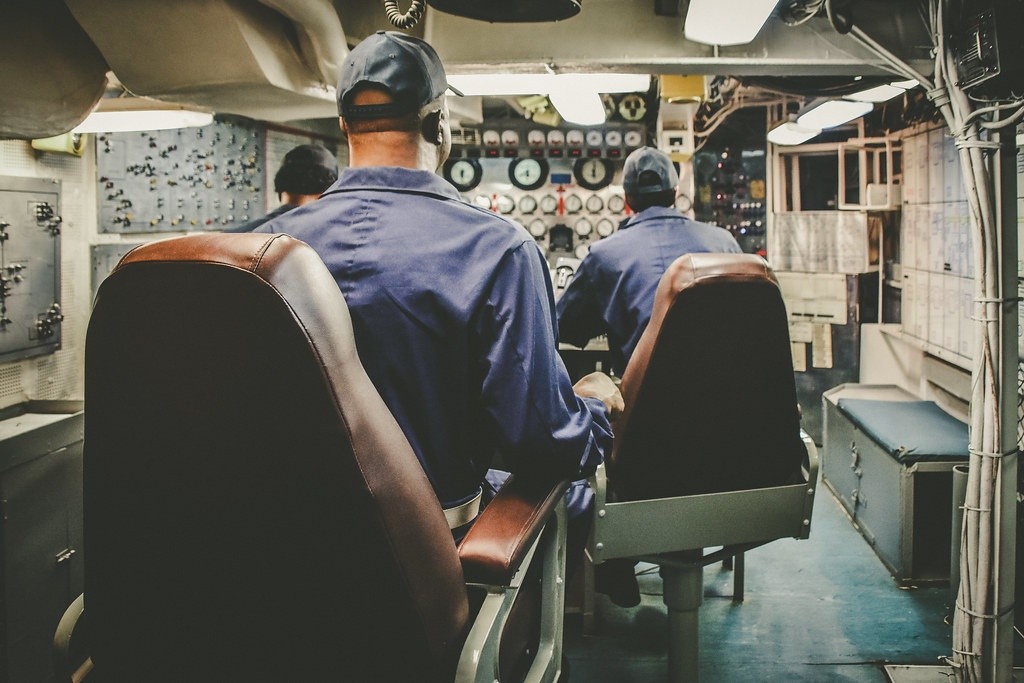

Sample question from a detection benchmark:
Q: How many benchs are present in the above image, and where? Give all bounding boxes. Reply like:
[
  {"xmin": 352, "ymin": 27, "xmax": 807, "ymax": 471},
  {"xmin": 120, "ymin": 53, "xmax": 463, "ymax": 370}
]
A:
[{"xmin": 821, "ymin": 382, "xmax": 970, "ymax": 587}]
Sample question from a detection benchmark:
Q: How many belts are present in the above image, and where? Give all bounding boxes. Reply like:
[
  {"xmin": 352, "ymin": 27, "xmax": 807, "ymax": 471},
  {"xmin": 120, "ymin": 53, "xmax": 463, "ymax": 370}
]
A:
[{"xmin": 442, "ymin": 487, "xmax": 485, "ymax": 529}]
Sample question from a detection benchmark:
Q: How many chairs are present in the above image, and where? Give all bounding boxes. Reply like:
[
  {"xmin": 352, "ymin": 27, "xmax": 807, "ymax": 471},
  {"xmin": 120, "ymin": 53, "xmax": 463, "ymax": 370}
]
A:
[
  {"xmin": 53, "ymin": 232, "xmax": 597, "ymax": 683},
  {"xmin": 583, "ymin": 252, "xmax": 821, "ymax": 683}
]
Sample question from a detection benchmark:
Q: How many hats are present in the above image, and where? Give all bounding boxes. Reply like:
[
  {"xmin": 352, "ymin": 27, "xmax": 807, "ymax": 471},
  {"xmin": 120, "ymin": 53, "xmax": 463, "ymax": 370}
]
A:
[
  {"xmin": 336, "ymin": 30, "xmax": 464, "ymax": 118},
  {"xmin": 275, "ymin": 144, "xmax": 339, "ymax": 195},
  {"xmin": 623, "ymin": 146, "xmax": 677, "ymax": 193}
]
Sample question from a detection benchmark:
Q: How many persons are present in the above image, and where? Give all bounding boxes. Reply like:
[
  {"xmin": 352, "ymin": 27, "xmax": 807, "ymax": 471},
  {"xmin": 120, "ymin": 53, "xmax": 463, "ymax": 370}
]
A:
[
  {"xmin": 225, "ymin": 141, "xmax": 337, "ymax": 234},
  {"xmin": 557, "ymin": 144, "xmax": 748, "ymax": 609},
  {"xmin": 249, "ymin": 29, "xmax": 626, "ymax": 683}
]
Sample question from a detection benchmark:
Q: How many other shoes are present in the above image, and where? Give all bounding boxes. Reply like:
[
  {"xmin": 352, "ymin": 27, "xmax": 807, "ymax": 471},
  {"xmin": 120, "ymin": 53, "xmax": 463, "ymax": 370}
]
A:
[{"xmin": 595, "ymin": 561, "xmax": 640, "ymax": 605}]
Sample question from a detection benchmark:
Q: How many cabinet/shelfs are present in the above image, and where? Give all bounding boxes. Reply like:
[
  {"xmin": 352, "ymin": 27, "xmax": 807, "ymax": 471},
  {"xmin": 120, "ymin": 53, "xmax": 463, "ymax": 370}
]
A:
[{"xmin": 0, "ymin": 440, "xmax": 83, "ymax": 683}]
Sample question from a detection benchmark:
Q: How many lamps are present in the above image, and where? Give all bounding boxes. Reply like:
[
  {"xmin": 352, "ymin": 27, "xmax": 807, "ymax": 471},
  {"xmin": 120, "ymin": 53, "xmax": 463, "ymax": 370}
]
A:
[{"xmin": 766, "ymin": 79, "xmax": 918, "ymax": 146}]
